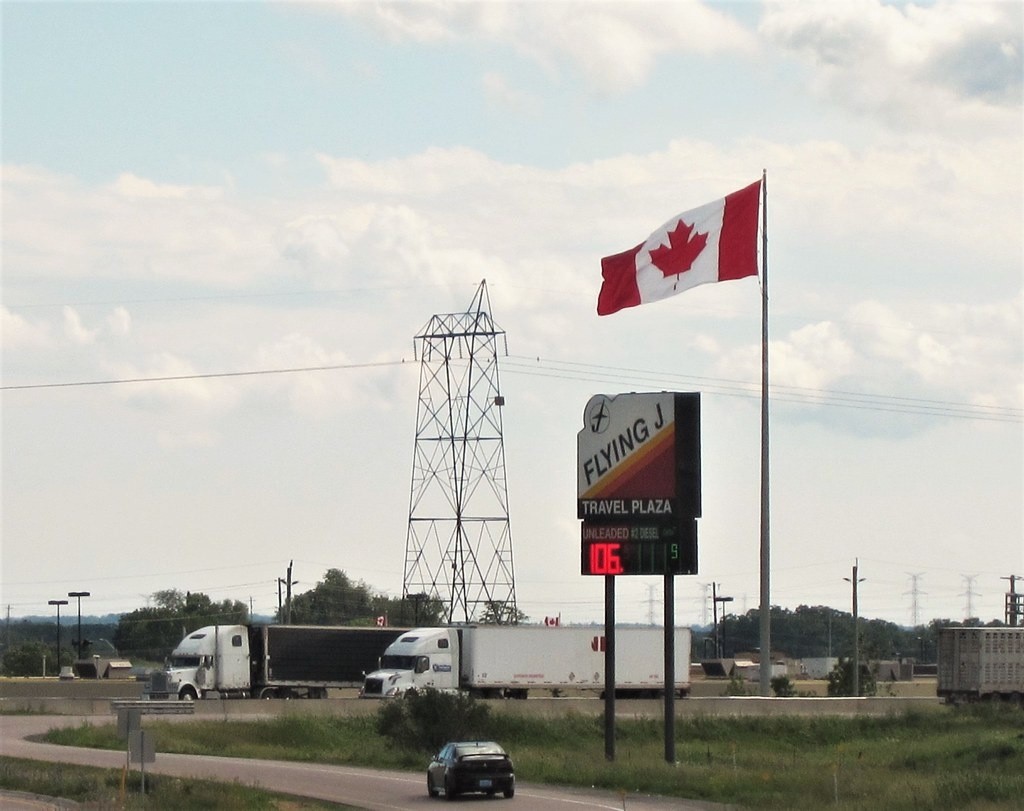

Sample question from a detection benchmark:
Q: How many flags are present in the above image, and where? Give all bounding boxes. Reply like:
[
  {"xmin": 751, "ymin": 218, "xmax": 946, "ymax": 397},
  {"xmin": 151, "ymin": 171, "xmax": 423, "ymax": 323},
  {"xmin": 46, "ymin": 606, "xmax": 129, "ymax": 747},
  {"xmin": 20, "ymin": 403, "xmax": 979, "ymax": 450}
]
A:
[{"xmin": 597, "ymin": 180, "xmax": 764, "ymax": 315}]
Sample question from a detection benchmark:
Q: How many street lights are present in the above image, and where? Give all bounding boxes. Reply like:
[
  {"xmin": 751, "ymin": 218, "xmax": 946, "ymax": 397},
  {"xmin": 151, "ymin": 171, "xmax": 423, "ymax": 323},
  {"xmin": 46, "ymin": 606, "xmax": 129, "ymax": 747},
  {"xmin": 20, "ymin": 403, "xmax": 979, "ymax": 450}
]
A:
[
  {"xmin": 708, "ymin": 582, "xmax": 733, "ymax": 660},
  {"xmin": 275, "ymin": 568, "xmax": 299, "ymax": 626},
  {"xmin": 843, "ymin": 566, "xmax": 866, "ymax": 697},
  {"xmin": 6, "ymin": 592, "xmax": 91, "ymax": 676}
]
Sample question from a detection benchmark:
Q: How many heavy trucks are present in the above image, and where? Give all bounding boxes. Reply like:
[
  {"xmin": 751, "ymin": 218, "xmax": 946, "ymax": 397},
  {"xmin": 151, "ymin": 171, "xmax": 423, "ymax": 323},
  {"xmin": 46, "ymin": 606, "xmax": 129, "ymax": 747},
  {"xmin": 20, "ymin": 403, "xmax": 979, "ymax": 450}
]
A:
[{"xmin": 165, "ymin": 625, "xmax": 690, "ymax": 700}]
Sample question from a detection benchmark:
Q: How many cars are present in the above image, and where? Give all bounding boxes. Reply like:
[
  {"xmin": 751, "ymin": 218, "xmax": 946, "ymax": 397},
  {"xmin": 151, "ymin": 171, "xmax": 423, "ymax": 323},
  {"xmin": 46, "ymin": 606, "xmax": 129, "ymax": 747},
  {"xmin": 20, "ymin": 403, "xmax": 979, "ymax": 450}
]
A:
[{"xmin": 427, "ymin": 742, "xmax": 515, "ymax": 801}]
